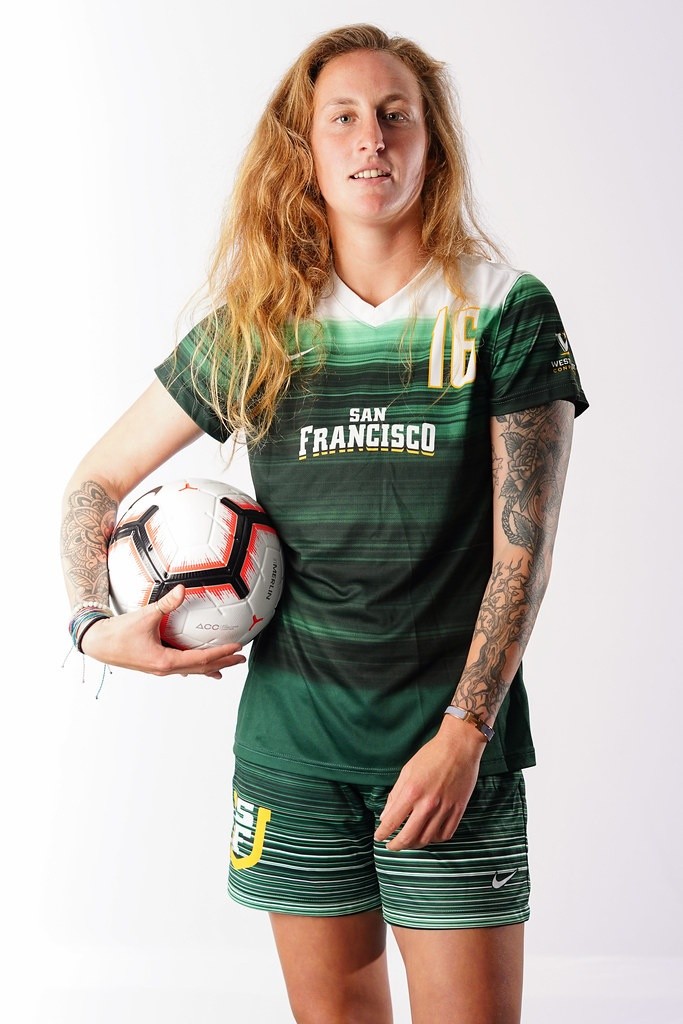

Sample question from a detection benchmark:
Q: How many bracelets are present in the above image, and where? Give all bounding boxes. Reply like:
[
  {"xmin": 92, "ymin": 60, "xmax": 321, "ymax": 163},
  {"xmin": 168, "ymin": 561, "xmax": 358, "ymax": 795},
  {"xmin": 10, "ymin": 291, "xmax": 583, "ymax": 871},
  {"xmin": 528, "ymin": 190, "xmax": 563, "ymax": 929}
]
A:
[
  {"xmin": 445, "ymin": 704, "xmax": 495, "ymax": 741},
  {"xmin": 69, "ymin": 601, "xmax": 112, "ymax": 653}
]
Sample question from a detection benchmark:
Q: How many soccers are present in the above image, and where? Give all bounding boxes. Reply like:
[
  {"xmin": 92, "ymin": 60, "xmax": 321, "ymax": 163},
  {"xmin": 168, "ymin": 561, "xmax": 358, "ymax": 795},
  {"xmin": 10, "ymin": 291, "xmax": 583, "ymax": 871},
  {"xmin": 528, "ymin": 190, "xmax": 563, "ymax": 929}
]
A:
[{"xmin": 108, "ymin": 477, "xmax": 284, "ymax": 650}]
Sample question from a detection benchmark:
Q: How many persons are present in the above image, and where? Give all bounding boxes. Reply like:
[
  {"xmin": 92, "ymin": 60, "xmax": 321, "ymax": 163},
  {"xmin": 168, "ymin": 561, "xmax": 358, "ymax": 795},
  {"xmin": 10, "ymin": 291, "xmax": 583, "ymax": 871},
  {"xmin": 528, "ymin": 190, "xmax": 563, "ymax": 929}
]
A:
[{"xmin": 60, "ymin": 25, "xmax": 590, "ymax": 1024}]
